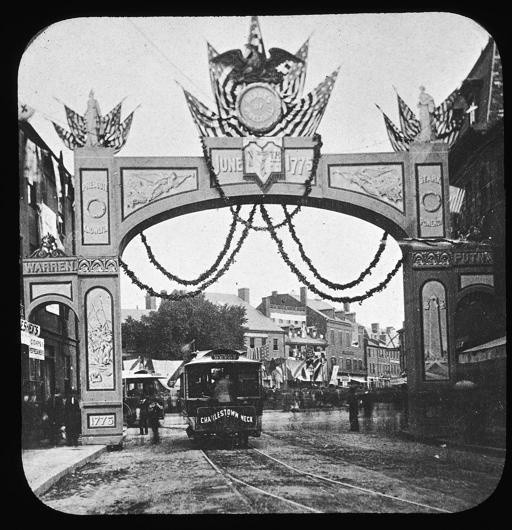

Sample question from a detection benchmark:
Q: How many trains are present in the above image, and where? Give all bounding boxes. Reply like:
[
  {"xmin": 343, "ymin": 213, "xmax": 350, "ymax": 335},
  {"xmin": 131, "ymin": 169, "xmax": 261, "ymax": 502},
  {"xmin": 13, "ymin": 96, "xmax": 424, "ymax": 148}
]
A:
[
  {"xmin": 178, "ymin": 348, "xmax": 263, "ymax": 449},
  {"xmin": 124, "ymin": 370, "xmax": 171, "ymax": 428}
]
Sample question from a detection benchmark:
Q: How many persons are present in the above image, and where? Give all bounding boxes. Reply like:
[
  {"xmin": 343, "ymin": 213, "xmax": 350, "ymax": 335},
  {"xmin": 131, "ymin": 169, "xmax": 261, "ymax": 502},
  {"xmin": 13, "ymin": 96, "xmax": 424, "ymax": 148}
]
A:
[
  {"xmin": 208, "ymin": 369, "xmax": 233, "ymax": 405},
  {"xmin": 21, "ymin": 380, "xmax": 182, "ymax": 451},
  {"xmin": 344, "ymin": 386, "xmax": 361, "ymax": 433}
]
